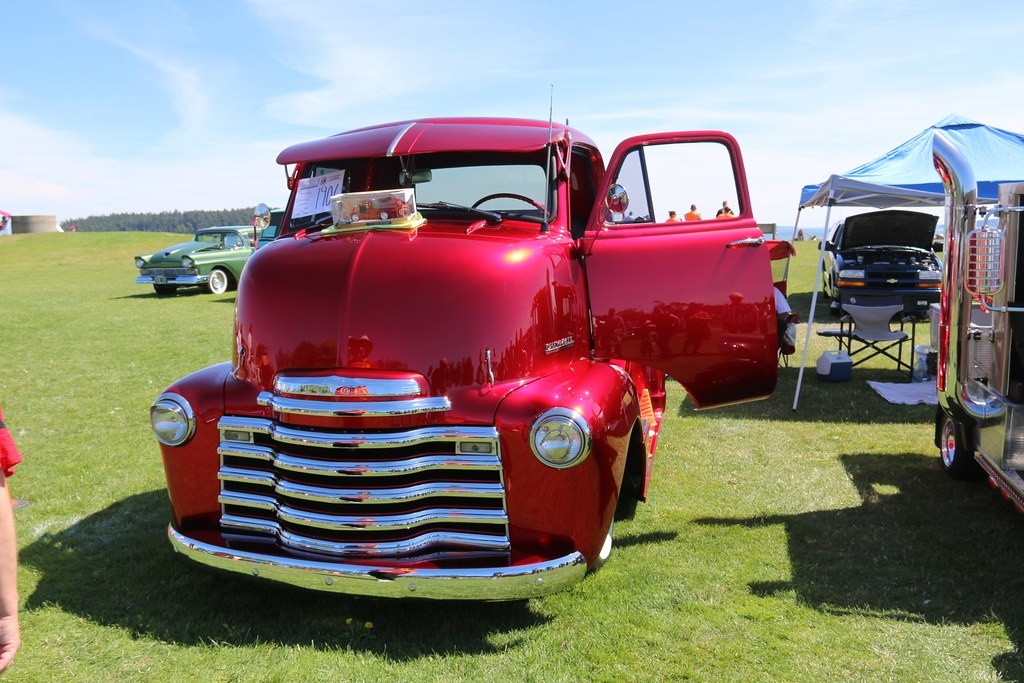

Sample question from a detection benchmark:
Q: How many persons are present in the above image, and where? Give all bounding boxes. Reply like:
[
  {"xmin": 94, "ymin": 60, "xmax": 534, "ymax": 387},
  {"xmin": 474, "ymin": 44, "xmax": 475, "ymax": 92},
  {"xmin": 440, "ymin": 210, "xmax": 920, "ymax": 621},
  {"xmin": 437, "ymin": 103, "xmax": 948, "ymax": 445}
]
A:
[
  {"xmin": 427, "ymin": 328, "xmax": 531, "ymax": 397},
  {"xmin": 235, "ymin": 323, "xmax": 253, "ymax": 382},
  {"xmin": 0, "ymin": 405, "xmax": 22, "ymax": 671},
  {"xmin": 773, "ymin": 286, "xmax": 796, "ymax": 355},
  {"xmin": 624, "ymin": 211, "xmax": 635, "ymax": 221},
  {"xmin": 717, "ymin": 201, "xmax": 734, "ymax": 215},
  {"xmin": 665, "ymin": 210, "xmax": 682, "ymax": 223},
  {"xmin": 601, "ymin": 292, "xmax": 760, "ymax": 363},
  {"xmin": 684, "ymin": 204, "xmax": 702, "ymax": 221},
  {"xmin": 717, "ymin": 206, "xmax": 733, "ymax": 218}
]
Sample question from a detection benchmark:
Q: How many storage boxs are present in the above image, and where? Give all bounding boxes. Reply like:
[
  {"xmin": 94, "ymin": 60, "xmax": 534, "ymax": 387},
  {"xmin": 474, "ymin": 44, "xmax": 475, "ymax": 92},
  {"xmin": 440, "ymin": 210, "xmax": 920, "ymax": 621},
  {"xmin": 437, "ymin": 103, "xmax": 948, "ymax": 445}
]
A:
[
  {"xmin": 929, "ymin": 300, "xmax": 992, "ymax": 381},
  {"xmin": 329, "ymin": 188, "xmax": 418, "ymax": 226}
]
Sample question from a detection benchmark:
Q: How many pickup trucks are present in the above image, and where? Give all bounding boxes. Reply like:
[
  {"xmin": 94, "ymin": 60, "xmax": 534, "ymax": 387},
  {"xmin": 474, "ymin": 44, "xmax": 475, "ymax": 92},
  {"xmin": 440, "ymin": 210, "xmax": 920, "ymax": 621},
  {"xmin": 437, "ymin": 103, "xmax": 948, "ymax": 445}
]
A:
[{"xmin": 135, "ymin": 226, "xmax": 277, "ymax": 294}]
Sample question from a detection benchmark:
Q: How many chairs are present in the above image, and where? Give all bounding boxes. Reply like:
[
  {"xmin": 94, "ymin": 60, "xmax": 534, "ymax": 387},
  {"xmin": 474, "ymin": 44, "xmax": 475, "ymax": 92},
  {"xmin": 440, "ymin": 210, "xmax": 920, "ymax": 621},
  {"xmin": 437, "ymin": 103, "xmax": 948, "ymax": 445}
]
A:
[
  {"xmin": 773, "ymin": 279, "xmax": 801, "ymax": 373},
  {"xmin": 840, "ymin": 301, "xmax": 913, "ymax": 377}
]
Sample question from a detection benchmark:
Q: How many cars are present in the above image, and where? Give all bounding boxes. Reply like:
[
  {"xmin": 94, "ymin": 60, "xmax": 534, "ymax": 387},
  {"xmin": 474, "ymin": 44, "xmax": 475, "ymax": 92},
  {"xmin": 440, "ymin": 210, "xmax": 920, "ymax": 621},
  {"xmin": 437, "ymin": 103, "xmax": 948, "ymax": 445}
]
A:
[
  {"xmin": 150, "ymin": 116, "xmax": 778, "ymax": 605},
  {"xmin": 819, "ymin": 210, "xmax": 944, "ymax": 315}
]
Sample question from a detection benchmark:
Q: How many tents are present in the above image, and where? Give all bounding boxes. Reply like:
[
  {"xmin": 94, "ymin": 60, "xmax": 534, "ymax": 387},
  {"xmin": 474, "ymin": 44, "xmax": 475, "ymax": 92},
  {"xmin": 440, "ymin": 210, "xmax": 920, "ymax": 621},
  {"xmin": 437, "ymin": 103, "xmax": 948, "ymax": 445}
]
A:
[{"xmin": 782, "ymin": 112, "xmax": 1024, "ymax": 412}]
